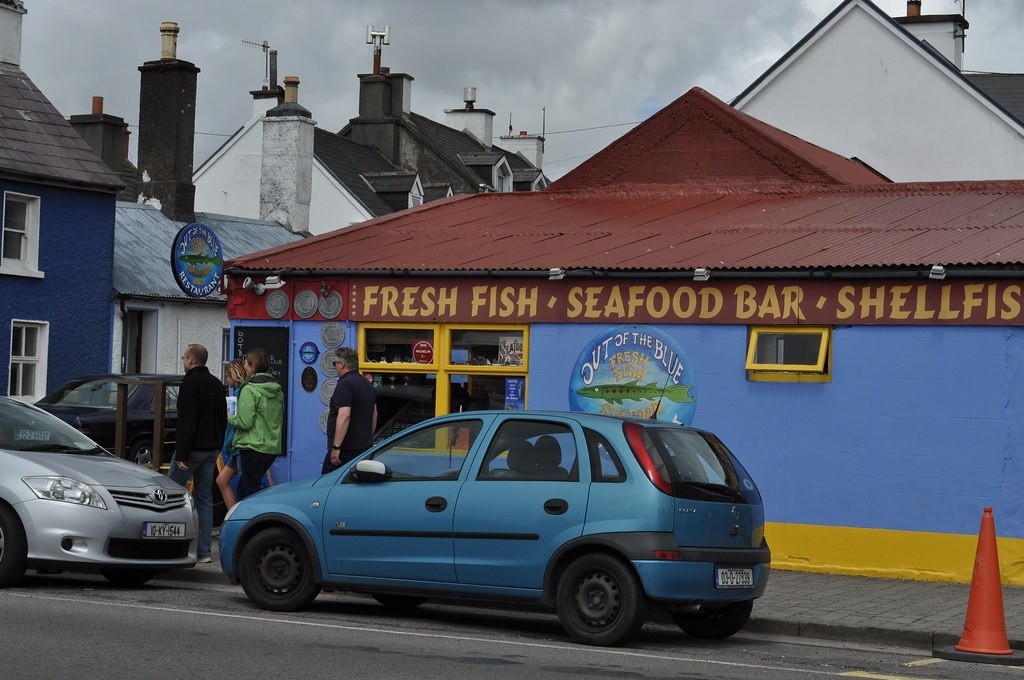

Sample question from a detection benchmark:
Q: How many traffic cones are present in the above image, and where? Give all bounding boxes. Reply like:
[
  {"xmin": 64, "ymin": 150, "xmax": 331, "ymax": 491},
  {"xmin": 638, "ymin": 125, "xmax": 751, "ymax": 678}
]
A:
[{"xmin": 933, "ymin": 507, "xmax": 1024, "ymax": 666}]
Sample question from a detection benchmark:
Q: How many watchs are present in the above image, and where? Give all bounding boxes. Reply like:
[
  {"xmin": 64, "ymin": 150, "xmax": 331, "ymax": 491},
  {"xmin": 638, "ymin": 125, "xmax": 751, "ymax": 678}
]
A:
[{"xmin": 333, "ymin": 445, "xmax": 341, "ymax": 450}]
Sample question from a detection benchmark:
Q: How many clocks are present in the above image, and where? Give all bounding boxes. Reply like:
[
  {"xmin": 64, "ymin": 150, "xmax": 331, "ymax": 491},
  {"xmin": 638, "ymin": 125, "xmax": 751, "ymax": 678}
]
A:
[{"xmin": 300, "ymin": 342, "xmax": 318, "ymax": 363}]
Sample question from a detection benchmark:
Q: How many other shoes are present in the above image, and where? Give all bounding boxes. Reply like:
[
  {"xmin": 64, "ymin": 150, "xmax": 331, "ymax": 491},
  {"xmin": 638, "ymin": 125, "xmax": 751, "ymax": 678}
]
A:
[{"xmin": 198, "ymin": 557, "xmax": 212, "ymax": 563}]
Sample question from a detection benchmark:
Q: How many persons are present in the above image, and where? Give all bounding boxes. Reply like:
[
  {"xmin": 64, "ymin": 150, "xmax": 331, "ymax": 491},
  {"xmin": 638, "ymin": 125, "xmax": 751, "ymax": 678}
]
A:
[
  {"xmin": 167, "ymin": 344, "xmax": 227, "ymax": 563},
  {"xmin": 216, "ymin": 347, "xmax": 285, "ymax": 511},
  {"xmin": 321, "ymin": 347, "xmax": 378, "ymax": 474}
]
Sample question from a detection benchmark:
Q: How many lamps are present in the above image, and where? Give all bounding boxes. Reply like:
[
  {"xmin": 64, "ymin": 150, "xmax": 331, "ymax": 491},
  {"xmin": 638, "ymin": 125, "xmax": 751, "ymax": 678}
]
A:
[
  {"xmin": 548, "ymin": 268, "xmax": 566, "ymax": 279},
  {"xmin": 243, "ymin": 277, "xmax": 266, "ymax": 296},
  {"xmin": 264, "ymin": 275, "xmax": 286, "ymax": 289},
  {"xmin": 928, "ymin": 266, "xmax": 946, "ymax": 280},
  {"xmin": 693, "ymin": 269, "xmax": 710, "ymax": 281}
]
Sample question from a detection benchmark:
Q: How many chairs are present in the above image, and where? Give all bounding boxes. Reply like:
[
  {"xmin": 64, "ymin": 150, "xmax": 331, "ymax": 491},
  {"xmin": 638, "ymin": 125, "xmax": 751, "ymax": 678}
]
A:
[{"xmin": 491, "ymin": 442, "xmax": 537, "ymax": 480}]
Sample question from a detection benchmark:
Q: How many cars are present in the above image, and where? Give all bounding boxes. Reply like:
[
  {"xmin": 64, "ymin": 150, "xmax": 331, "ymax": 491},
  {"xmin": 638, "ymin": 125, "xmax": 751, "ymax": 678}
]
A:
[
  {"xmin": 33, "ymin": 373, "xmax": 188, "ymax": 468},
  {"xmin": 1, "ymin": 397, "xmax": 200, "ymax": 587},
  {"xmin": 218, "ymin": 410, "xmax": 770, "ymax": 646}
]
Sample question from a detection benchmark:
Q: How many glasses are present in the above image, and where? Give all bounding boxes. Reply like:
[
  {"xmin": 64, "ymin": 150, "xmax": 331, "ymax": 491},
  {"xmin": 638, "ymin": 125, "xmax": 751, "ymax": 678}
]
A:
[{"xmin": 333, "ymin": 362, "xmax": 342, "ymax": 366}]
